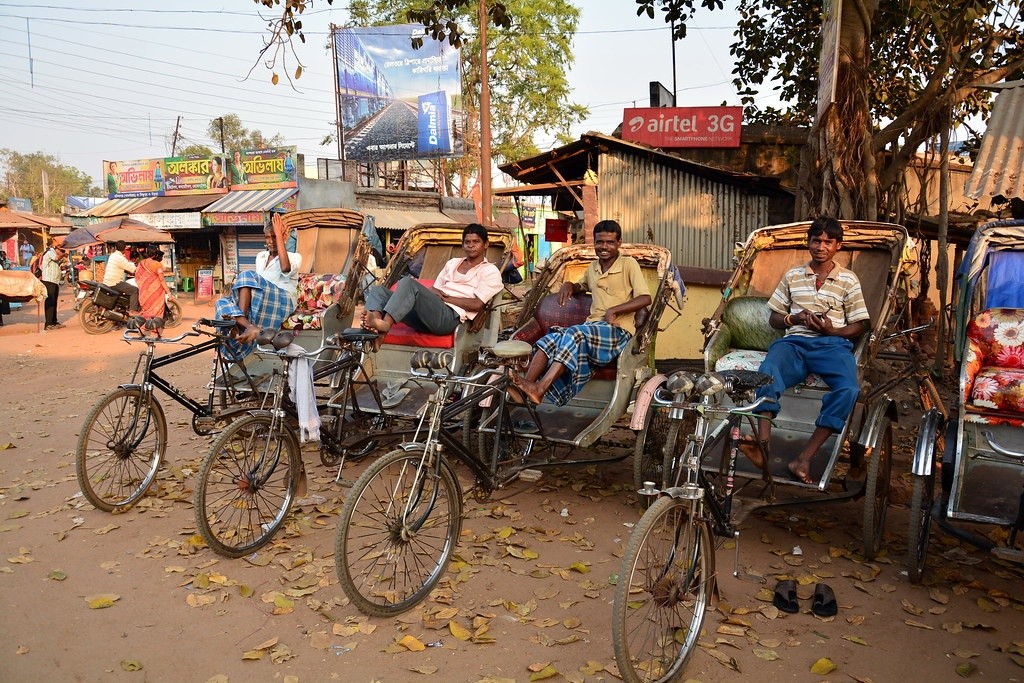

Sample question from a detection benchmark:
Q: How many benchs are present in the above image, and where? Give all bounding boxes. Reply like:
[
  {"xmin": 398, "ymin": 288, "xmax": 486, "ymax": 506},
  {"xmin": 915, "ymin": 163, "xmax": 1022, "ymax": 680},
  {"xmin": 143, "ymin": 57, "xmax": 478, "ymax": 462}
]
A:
[
  {"xmin": 370, "ymin": 276, "xmax": 458, "ymax": 347},
  {"xmin": 704, "ymin": 296, "xmax": 833, "ymax": 395},
  {"xmin": 506, "ymin": 292, "xmax": 650, "ymax": 377},
  {"xmin": 961, "ymin": 309, "xmax": 1024, "ymax": 417},
  {"xmin": 281, "ymin": 272, "xmax": 347, "ymax": 330}
]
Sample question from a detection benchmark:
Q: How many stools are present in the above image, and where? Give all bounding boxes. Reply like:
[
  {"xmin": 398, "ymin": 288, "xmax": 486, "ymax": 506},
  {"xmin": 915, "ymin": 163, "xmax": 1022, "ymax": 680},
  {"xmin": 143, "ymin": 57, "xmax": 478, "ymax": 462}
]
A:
[{"xmin": 179, "ymin": 277, "xmax": 195, "ymax": 292}]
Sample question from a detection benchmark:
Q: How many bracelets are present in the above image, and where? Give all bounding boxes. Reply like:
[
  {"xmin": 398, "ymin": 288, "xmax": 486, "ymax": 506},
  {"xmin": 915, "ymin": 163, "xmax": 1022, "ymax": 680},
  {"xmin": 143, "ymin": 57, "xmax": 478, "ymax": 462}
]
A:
[
  {"xmin": 787, "ymin": 314, "xmax": 793, "ymax": 325},
  {"xmin": 784, "ymin": 314, "xmax": 792, "ymax": 326}
]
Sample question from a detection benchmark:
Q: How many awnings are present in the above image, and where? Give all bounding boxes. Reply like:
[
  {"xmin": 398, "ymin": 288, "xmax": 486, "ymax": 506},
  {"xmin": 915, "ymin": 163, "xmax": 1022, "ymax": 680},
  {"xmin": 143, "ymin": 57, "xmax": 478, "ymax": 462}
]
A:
[
  {"xmin": 359, "ymin": 204, "xmax": 458, "ymax": 229},
  {"xmin": 62, "ymin": 196, "xmax": 157, "ymax": 228},
  {"xmin": 127, "ymin": 192, "xmax": 225, "ymax": 229},
  {"xmin": 201, "ymin": 187, "xmax": 300, "ymax": 227},
  {"xmin": 441, "ymin": 208, "xmax": 530, "ymax": 229}
]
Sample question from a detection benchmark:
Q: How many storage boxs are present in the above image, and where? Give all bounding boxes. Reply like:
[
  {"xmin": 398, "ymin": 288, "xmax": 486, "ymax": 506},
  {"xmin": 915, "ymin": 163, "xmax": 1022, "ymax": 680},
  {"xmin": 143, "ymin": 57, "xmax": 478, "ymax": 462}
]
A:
[{"xmin": 92, "ymin": 283, "xmax": 118, "ymax": 309}]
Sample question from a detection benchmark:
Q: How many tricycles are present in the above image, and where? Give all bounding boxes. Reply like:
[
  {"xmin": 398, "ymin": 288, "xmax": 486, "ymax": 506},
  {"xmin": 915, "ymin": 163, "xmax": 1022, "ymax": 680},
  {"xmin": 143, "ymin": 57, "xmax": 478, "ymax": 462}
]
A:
[
  {"xmin": 195, "ymin": 221, "xmax": 521, "ymax": 561},
  {"xmin": 332, "ymin": 240, "xmax": 689, "ymax": 618},
  {"xmin": 905, "ymin": 219, "xmax": 1024, "ymax": 581},
  {"xmin": 608, "ymin": 217, "xmax": 924, "ymax": 683},
  {"xmin": 76, "ymin": 210, "xmax": 390, "ymax": 512}
]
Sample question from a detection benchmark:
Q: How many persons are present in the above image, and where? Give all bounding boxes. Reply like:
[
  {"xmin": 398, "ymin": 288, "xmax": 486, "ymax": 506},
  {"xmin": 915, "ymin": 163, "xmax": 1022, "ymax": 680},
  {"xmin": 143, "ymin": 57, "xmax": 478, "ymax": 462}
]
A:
[
  {"xmin": 360, "ymin": 223, "xmax": 505, "ymax": 353},
  {"xmin": 103, "ymin": 240, "xmax": 141, "ymax": 317},
  {"xmin": 40, "ymin": 238, "xmax": 68, "ymax": 330},
  {"xmin": 501, "ymin": 250, "xmax": 523, "ymax": 284},
  {"xmin": 107, "ymin": 151, "xmax": 300, "ymax": 195},
  {"xmin": 496, "ymin": 219, "xmax": 653, "ymax": 404},
  {"xmin": 67, "ymin": 256, "xmax": 91, "ymax": 283},
  {"xmin": 130, "ymin": 246, "xmax": 174, "ymax": 321},
  {"xmin": 214, "ymin": 211, "xmax": 302, "ymax": 361},
  {"xmin": 18, "ymin": 239, "xmax": 35, "ymax": 267},
  {"xmin": 361, "ymin": 242, "xmax": 425, "ymax": 302},
  {"xmin": 739, "ymin": 216, "xmax": 872, "ymax": 484}
]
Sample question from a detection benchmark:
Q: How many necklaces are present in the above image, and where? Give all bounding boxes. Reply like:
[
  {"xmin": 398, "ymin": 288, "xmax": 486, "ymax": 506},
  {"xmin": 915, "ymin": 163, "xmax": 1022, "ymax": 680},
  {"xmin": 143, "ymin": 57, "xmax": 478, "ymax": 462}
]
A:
[{"xmin": 811, "ymin": 261, "xmax": 832, "ymax": 274}]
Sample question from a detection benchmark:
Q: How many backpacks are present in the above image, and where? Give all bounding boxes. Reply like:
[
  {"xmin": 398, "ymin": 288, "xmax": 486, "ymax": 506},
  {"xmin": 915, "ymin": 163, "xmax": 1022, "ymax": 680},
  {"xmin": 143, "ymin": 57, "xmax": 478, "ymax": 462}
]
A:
[{"xmin": 30, "ymin": 249, "xmax": 51, "ymax": 281}]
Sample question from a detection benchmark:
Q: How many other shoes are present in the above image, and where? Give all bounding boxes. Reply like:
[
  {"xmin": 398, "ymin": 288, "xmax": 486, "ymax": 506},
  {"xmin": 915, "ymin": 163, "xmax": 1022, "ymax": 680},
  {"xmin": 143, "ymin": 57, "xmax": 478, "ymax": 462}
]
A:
[
  {"xmin": 44, "ymin": 325, "xmax": 60, "ymax": 331},
  {"xmin": 56, "ymin": 323, "xmax": 66, "ymax": 328}
]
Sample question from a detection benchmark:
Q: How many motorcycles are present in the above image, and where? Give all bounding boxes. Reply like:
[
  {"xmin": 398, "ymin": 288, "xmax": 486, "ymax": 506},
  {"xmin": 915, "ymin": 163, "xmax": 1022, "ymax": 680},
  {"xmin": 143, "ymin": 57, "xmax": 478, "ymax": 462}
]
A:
[{"xmin": 75, "ymin": 274, "xmax": 183, "ymax": 334}]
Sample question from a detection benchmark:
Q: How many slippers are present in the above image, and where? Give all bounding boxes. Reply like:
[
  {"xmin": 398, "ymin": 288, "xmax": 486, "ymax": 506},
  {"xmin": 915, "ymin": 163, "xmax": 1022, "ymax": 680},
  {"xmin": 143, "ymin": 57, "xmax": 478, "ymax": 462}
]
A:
[
  {"xmin": 220, "ymin": 374, "xmax": 246, "ymax": 387},
  {"xmin": 811, "ymin": 583, "xmax": 838, "ymax": 617},
  {"xmin": 773, "ymin": 579, "xmax": 800, "ymax": 613},
  {"xmin": 520, "ymin": 420, "xmax": 544, "ymax": 434},
  {"xmin": 381, "ymin": 387, "xmax": 411, "ymax": 409},
  {"xmin": 511, "ymin": 419, "xmax": 524, "ymax": 432},
  {"xmin": 381, "ymin": 377, "xmax": 409, "ymax": 398},
  {"xmin": 242, "ymin": 372, "xmax": 271, "ymax": 388}
]
